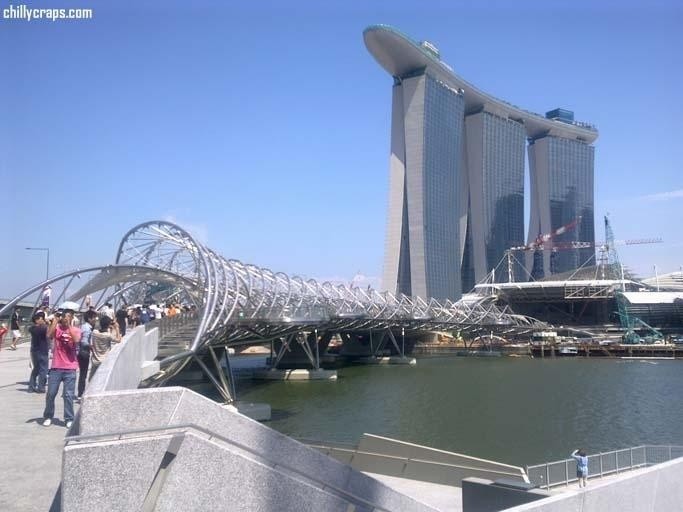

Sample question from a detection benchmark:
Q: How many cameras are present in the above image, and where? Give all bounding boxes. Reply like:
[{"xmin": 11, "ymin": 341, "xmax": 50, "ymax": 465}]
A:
[{"xmin": 55, "ymin": 312, "xmax": 63, "ymax": 317}]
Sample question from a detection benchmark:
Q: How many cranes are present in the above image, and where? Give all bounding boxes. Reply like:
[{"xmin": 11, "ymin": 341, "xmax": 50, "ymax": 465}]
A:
[{"xmin": 506, "ymin": 215, "xmax": 664, "ymax": 343}]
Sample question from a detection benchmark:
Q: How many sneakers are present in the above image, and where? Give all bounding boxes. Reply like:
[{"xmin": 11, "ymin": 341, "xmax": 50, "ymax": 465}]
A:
[
  {"xmin": 43, "ymin": 419, "xmax": 52, "ymax": 426},
  {"xmin": 66, "ymin": 421, "xmax": 72, "ymax": 428},
  {"xmin": 11, "ymin": 345, "xmax": 16, "ymax": 350}
]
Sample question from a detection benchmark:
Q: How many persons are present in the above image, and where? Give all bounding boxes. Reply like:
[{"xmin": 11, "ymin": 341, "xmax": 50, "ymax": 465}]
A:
[
  {"xmin": 571, "ymin": 449, "xmax": 588, "ymax": 488},
  {"xmin": 0, "ymin": 302, "xmax": 196, "ymax": 428}
]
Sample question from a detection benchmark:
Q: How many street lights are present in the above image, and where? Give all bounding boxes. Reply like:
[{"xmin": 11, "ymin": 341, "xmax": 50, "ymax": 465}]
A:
[{"xmin": 25, "ymin": 247, "xmax": 49, "ymax": 279}]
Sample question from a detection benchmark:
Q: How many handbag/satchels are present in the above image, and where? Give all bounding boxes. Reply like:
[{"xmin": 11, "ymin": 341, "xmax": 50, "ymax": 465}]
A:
[{"xmin": 577, "ymin": 471, "xmax": 582, "ymax": 477}]
